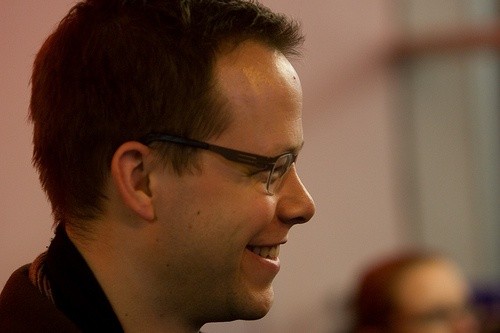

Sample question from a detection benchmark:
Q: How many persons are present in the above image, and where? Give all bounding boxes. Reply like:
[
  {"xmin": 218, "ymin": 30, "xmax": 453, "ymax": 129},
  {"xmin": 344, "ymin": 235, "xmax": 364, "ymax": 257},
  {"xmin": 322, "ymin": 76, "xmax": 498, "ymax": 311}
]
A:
[
  {"xmin": 346, "ymin": 245, "xmax": 484, "ymax": 333},
  {"xmin": 0, "ymin": 0, "xmax": 317, "ymax": 333}
]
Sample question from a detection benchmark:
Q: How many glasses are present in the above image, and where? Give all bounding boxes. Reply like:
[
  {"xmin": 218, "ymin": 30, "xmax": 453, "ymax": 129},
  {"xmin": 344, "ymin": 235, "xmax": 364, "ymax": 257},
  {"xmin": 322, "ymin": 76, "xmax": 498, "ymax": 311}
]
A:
[{"xmin": 145, "ymin": 131, "xmax": 298, "ymax": 193}]
